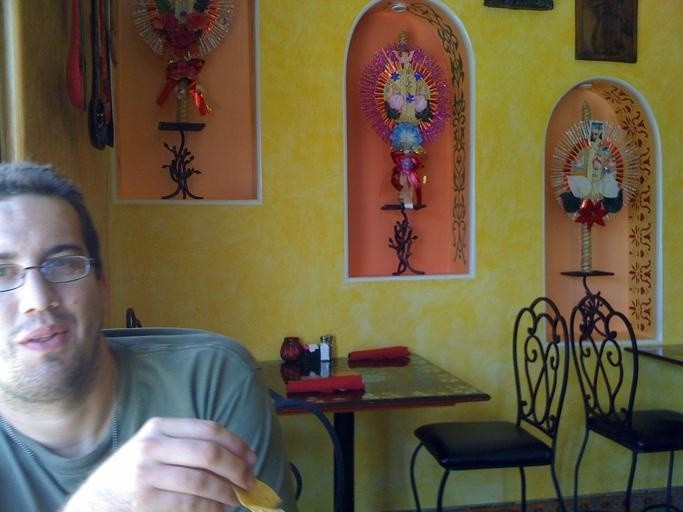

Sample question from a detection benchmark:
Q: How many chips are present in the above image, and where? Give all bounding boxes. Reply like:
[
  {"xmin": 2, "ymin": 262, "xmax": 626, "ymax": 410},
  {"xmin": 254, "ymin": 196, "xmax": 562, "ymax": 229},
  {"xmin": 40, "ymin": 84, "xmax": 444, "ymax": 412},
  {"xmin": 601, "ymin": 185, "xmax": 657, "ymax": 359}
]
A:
[{"xmin": 232, "ymin": 479, "xmax": 285, "ymax": 512}]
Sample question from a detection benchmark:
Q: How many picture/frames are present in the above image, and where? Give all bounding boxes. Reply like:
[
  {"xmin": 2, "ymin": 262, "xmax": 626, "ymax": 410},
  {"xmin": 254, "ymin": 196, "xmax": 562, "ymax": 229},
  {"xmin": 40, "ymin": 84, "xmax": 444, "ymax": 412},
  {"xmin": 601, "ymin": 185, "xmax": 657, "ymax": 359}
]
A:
[
  {"xmin": 575, "ymin": 0, "xmax": 639, "ymax": 64},
  {"xmin": 483, "ymin": 0, "xmax": 555, "ymax": 11}
]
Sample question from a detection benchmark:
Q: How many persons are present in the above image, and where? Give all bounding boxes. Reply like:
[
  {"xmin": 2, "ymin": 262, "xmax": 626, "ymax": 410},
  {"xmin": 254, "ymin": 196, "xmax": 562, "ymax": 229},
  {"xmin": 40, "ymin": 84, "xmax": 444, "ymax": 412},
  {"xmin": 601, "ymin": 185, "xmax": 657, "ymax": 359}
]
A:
[{"xmin": 2, "ymin": 160, "xmax": 299, "ymax": 507}]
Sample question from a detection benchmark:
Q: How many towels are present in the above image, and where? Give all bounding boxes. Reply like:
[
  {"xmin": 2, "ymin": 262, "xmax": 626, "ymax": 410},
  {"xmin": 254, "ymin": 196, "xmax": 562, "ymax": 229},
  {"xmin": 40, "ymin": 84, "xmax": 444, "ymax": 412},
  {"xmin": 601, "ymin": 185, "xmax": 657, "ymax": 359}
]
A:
[
  {"xmin": 283, "ymin": 373, "xmax": 366, "ymax": 395},
  {"xmin": 350, "ymin": 347, "xmax": 411, "ymax": 362}
]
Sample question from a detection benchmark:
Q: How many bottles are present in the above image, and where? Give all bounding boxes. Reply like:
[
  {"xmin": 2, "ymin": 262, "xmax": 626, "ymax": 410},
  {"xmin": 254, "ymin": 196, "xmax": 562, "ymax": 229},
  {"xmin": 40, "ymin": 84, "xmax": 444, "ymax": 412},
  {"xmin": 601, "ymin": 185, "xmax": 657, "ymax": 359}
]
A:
[
  {"xmin": 280, "ymin": 337, "xmax": 301, "ymax": 363},
  {"xmin": 320, "ymin": 336, "xmax": 331, "ymax": 362}
]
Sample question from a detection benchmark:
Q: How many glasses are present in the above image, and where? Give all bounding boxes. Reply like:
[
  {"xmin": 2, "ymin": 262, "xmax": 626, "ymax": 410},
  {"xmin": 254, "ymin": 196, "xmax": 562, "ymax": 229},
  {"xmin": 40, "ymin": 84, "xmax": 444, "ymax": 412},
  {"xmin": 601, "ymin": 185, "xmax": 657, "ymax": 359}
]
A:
[{"xmin": 0, "ymin": 256, "xmax": 96, "ymax": 292}]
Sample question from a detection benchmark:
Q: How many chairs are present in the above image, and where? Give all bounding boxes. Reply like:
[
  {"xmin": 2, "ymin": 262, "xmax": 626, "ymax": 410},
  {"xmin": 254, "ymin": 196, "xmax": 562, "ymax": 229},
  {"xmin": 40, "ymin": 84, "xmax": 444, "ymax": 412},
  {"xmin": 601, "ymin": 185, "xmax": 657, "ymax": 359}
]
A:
[
  {"xmin": 125, "ymin": 307, "xmax": 144, "ymax": 328},
  {"xmin": 409, "ymin": 297, "xmax": 570, "ymax": 511},
  {"xmin": 264, "ymin": 386, "xmax": 349, "ymax": 511},
  {"xmin": 569, "ymin": 292, "xmax": 683, "ymax": 512}
]
